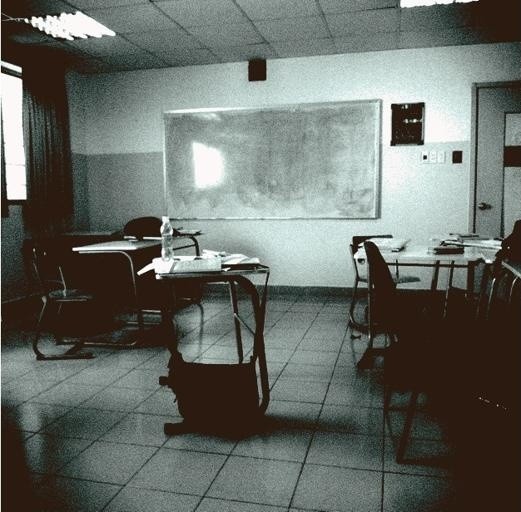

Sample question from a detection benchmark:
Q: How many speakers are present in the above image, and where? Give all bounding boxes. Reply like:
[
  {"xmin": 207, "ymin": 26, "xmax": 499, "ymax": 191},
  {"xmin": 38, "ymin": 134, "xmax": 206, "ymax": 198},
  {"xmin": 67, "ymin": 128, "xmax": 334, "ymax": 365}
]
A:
[
  {"xmin": 390, "ymin": 101, "xmax": 425, "ymax": 146},
  {"xmin": 248, "ymin": 57, "xmax": 267, "ymax": 81}
]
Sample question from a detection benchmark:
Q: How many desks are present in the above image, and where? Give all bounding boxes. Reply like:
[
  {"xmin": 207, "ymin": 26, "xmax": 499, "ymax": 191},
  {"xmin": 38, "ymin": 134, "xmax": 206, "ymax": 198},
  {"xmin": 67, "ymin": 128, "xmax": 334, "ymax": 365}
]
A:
[{"xmin": 68, "ymin": 228, "xmax": 272, "ymax": 417}]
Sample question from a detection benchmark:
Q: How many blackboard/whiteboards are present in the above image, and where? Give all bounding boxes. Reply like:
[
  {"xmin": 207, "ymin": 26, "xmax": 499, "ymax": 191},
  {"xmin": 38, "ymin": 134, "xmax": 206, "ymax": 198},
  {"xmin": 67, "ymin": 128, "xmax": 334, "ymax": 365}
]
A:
[{"xmin": 163, "ymin": 98, "xmax": 383, "ymax": 220}]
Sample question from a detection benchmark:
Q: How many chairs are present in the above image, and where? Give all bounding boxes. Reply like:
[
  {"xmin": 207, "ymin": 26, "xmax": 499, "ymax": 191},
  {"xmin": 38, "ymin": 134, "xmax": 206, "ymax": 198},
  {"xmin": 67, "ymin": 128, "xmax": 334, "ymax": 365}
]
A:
[{"xmin": 20, "ymin": 217, "xmax": 168, "ymax": 360}]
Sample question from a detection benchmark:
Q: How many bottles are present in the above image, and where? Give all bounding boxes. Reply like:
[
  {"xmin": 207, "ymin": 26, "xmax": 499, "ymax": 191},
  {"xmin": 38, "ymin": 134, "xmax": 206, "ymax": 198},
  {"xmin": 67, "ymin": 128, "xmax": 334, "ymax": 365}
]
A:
[{"xmin": 161, "ymin": 215, "xmax": 175, "ymax": 261}]
